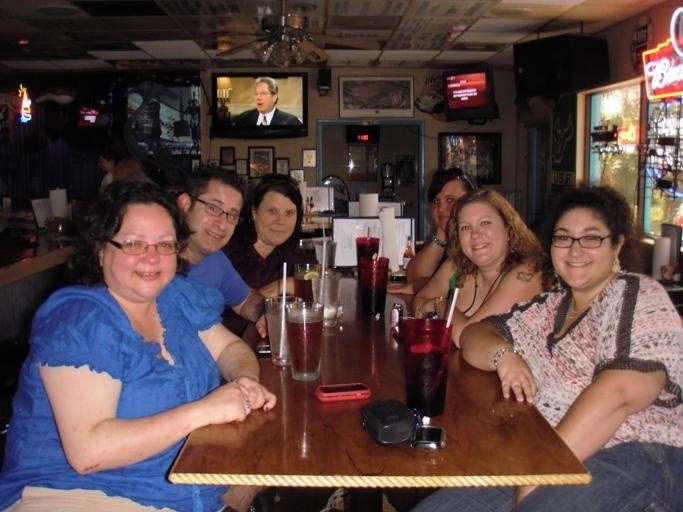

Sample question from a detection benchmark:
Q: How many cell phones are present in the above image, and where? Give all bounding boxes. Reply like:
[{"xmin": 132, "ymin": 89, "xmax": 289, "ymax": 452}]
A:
[
  {"xmin": 254, "ymin": 341, "xmax": 271, "ymax": 357},
  {"xmin": 315, "ymin": 383, "xmax": 372, "ymax": 402},
  {"xmin": 412, "ymin": 426, "xmax": 447, "ymax": 450}
]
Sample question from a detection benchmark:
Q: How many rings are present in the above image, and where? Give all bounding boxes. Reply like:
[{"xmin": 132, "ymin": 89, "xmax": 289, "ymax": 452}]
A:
[
  {"xmin": 415, "ymin": 307, "xmax": 420, "ymax": 312},
  {"xmin": 244, "ymin": 404, "xmax": 249, "ymax": 410}
]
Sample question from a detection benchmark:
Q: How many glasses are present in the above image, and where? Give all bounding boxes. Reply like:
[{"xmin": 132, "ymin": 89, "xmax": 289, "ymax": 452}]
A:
[
  {"xmin": 552, "ymin": 235, "xmax": 611, "ymax": 248},
  {"xmin": 109, "ymin": 239, "xmax": 181, "ymax": 255},
  {"xmin": 190, "ymin": 196, "xmax": 244, "ymax": 226},
  {"xmin": 433, "ymin": 168, "xmax": 464, "ymax": 182}
]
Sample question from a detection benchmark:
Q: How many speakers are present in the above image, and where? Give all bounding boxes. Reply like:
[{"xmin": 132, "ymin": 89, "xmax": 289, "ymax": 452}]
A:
[
  {"xmin": 318, "ymin": 68, "xmax": 331, "ymax": 91},
  {"xmin": 512, "ymin": 33, "xmax": 608, "ymax": 97}
]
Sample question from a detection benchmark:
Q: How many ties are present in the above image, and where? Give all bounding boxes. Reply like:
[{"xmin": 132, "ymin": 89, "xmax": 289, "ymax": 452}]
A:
[{"xmin": 259, "ymin": 116, "xmax": 267, "ymax": 125}]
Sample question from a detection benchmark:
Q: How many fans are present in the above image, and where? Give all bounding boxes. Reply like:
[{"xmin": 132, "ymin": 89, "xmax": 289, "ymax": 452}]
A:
[{"xmin": 205, "ymin": 0, "xmax": 380, "ymax": 69}]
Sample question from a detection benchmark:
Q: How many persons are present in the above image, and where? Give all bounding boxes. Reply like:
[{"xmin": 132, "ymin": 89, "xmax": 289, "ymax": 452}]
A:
[
  {"xmin": 56, "ymin": 121, "xmax": 158, "ymax": 193},
  {"xmin": 230, "ymin": 77, "xmax": 302, "ymax": 125},
  {"xmin": 404, "ymin": 166, "xmax": 479, "ymax": 289},
  {"xmin": 177, "ymin": 166, "xmax": 275, "ymax": 337},
  {"xmin": 222, "ymin": 172, "xmax": 313, "ymax": 334},
  {"xmin": 411, "ymin": 185, "xmax": 683, "ymax": 512},
  {"xmin": 412, "ymin": 191, "xmax": 545, "ymax": 349},
  {"xmin": 0, "ymin": 178, "xmax": 278, "ymax": 511}
]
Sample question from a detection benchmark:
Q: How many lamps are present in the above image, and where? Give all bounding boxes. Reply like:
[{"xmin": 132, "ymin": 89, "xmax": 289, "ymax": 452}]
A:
[
  {"xmin": 253, "ymin": 32, "xmax": 309, "ymax": 69},
  {"xmin": 321, "ymin": 175, "xmax": 350, "ymax": 202}
]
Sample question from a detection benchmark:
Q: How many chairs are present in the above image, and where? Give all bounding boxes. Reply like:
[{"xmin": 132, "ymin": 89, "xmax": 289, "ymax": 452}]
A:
[
  {"xmin": 332, "ymin": 216, "xmax": 417, "ymax": 269},
  {"xmin": 347, "ymin": 200, "xmax": 404, "ymax": 218},
  {"xmin": 301, "ymin": 188, "xmax": 336, "ymax": 213}
]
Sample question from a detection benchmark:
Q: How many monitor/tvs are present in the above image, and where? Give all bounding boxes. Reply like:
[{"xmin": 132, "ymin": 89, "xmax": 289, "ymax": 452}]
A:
[
  {"xmin": 78, "ymin": 107, "xmax": 100, "ymax": 127},
  {"xmin": 442, "ymin": 65, "xmax": 494, "ymax": 120},
  {"xmin": 212, "ymin": 71, "xmax": 308, "ymax": 139}
]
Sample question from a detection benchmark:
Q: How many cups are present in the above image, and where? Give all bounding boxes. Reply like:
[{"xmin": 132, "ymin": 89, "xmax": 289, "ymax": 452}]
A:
[
  {"xmin": 355, "ymin": 237, "xmax": 380, "ymax": 272},
  {"xmin": 358, "ymin": 318, "xmax": 390, "ymax": 381},
  {"xmin": 360, "ymin": 255, "xmax": 386, "ymax": 314},
  {"xmin": 284, "ymin": 301, "xmax": 326, "ymax": 383},
  {"xmin": 269, "ymin": 370, "xmax": 322, "ymax": 468},
  {"xmin": 318, "ymin": 269, "xmax": 341, "ymax": 328},
  {"xmin": 406, "ymin": 318, "xmax": 448, "ymax": 417},
  {"xmin": 263, "ymin": 296, "xmax": 303, "ymax": 367},
  {"xmin": 292, "ymin": 262, "xmax": 324, "ymax": 302}
]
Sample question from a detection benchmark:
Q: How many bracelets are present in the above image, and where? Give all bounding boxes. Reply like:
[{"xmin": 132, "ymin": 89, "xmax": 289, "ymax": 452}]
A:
[
  {"xmin": 434, "ymin": 296, "xmax": 447, "ymax": 309},
  {"xmin": 491, "ymin": 346, "xmax": 520, "ymax": 368},
  {"xmin": 431, "ymin": 234, "xmax": 446, "ymax": 248}
]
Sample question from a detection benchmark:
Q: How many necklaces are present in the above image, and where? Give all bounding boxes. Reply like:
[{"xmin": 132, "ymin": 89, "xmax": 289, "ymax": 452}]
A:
[{"xmin": 571, "ymin": 304, "xmax": 587, "ymax": 316}]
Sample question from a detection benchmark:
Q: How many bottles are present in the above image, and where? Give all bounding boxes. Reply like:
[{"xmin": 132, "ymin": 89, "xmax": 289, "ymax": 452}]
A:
[
  {"xmin": 306, "ymin": 195, "xmax": 314, "ymax": 213},
  {"xmin": 401, "ymin": 236, "xmax": 414, "ymax": 272}
]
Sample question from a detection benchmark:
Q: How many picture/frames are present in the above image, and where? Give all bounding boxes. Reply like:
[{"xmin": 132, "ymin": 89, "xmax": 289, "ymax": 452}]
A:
[
  {"xmin": 207, "ymin": 143, "xmax": 316, "ymax": 186},
  {"xmin": 337, "ymin": 74, "xmax": 416, "ymax": 119}
]
